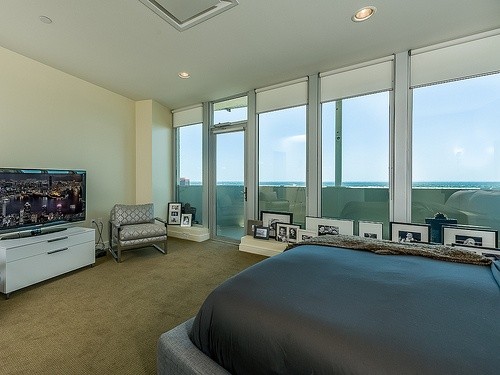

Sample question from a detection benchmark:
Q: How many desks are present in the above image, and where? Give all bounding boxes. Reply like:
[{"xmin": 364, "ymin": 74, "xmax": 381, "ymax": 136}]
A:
[{"xmin": 2, "ymin": 228, "xmax": 96, "ymax": 298}]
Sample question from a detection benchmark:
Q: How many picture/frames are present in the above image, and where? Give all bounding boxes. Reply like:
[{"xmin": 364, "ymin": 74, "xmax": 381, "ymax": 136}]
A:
[{"xmin": 168, "ymin": 203, "xmax": 498, "ymax": 249}]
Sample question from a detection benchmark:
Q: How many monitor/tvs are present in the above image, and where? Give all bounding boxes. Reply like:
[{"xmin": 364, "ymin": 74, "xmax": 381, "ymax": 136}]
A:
[{"xmin": 0, "ymin": 167, "xmax": 87, "ymax": 237}]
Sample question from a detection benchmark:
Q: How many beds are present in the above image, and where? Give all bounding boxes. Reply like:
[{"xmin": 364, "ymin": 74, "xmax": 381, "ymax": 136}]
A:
[{"xmin": 157, "ymin": 237, "xmax": 500, "ymax": 375}]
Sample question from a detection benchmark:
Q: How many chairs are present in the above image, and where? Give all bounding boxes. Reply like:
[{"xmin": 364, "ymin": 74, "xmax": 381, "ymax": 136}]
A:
[{"xmin": 108, "ymin": 203, "xmax": 167, "ymax": 262}]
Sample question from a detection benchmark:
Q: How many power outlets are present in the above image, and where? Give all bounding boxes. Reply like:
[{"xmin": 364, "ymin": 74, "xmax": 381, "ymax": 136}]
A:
[
  {"xmin": 91, "ymin": 219, "xmax": 95, "ymax": 227},
  {"xmin": 97, "ymin": 218, "xmax": 103, "ymax": 226}
]
